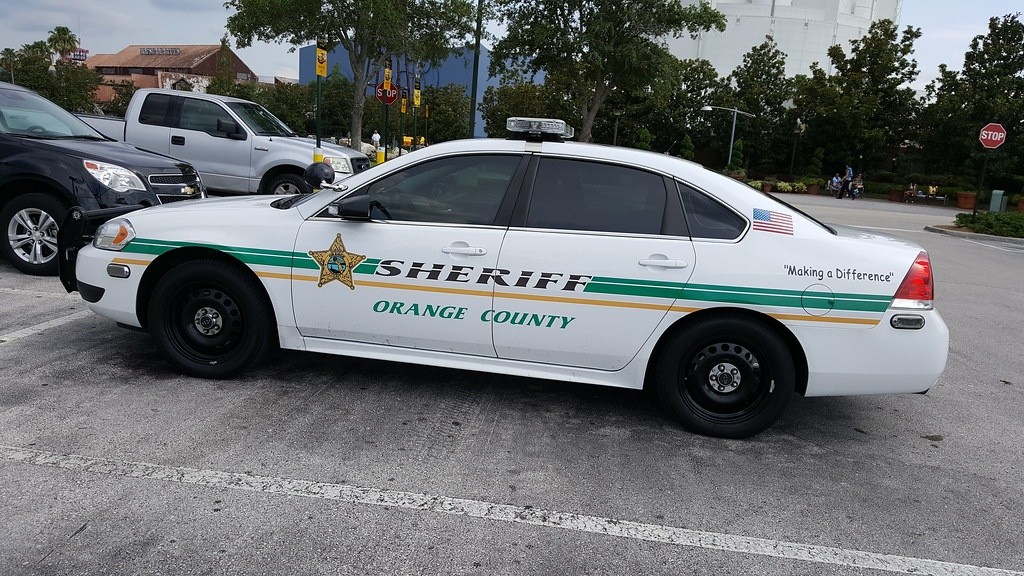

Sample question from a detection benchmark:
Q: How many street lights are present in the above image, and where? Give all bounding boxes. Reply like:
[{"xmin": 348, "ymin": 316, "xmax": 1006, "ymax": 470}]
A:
[{"xmin": 700, "ymin": 103, "xmax": 737, "ymax": 165}]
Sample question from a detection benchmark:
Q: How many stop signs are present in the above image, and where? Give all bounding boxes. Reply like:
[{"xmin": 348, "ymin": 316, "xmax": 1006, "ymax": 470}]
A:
[
  {"xmin": 376, "ymin": 81, "xmax": 398, "ymax": 106},
  {"xmin": 979, "ymin": 123, "xmax": 1006, "ymax": 149}
]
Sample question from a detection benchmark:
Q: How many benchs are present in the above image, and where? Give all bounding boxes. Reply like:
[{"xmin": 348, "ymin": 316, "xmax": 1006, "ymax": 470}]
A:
[
  {"xmin": 823, "ymin": 179, "xmax": 865, "ymax": 199},
  {"xmin": 903, "ymin": 190, "xmax": 949, "ymax": 207}
]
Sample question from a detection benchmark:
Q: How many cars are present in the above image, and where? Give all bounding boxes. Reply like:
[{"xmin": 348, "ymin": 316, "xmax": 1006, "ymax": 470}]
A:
[
  {"xmin": 54, "ymin": 136, "xmax": 948, "ymax": 439},
  {"xmin": 0, "ymin": 81, "xmax": 209, "ymax": 274}
]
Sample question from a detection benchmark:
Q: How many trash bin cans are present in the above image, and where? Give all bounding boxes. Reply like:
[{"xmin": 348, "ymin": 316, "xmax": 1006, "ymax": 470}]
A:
[{"xmin": 989, "ymin": 190, "xmax": 1008, "ymax": 213}]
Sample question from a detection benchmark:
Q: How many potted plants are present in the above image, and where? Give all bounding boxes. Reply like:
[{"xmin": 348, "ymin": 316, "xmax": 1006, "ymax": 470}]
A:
[
  {"xmin": 956, "ymin": 158, "xmax": 978, "ymax": 209},
  {"xmin": 883, "ymin": 184, "xmax": 907, "ymax": 202},
  {"xmin": 723, "ymin": 136, "xmax": 808, "ymax": 194},
  {"xmin": 803, "ymin": 147, "xmax": 825, "ymax": 194}
]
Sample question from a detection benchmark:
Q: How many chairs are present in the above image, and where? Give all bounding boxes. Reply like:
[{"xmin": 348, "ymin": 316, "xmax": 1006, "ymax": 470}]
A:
[
  {"xmin": 464, "ymin": 164, "xmax": 514, "ymax": 222},
  {"xmin": 536, "ymin": 176, "xmax": 585, "ymax": 226}
]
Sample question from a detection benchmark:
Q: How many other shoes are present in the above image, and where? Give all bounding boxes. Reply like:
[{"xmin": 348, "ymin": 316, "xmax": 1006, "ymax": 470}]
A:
[
  {"xmin": 852, "ymin": 194, "xmax": 855, "ymax": 200},
  {"xmin": 836, "ymin": 196, "xmax": 842, "ymax": 199}
]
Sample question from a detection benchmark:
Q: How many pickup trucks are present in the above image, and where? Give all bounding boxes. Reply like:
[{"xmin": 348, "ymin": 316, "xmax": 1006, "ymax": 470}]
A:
[{"xmin": 17, "ymin": 87, "xmax": 371, "ymax": 195}]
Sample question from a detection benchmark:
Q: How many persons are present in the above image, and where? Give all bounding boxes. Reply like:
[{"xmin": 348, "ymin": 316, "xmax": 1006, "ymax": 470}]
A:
[
  {"xmin": 836, "ymin": 162, "xmax": 857, "ymax": 200},
  {"xmin": 850, "ymin": 172, "xmax": 864, "ymax": 197},
  {"xmin": 370, "ymin": 130, "xmax": 381, "ymax": 148},
  {"xmin": 925, "ymin": 181, "xmax": 939, "ymax": 201},
  {"xmin": 905, "ymin": 179, "xmax": 918, "ymax": 204},
  {"xmin": 833, "ymin": 173, "xmax": 841, "ymax": 188}
]
